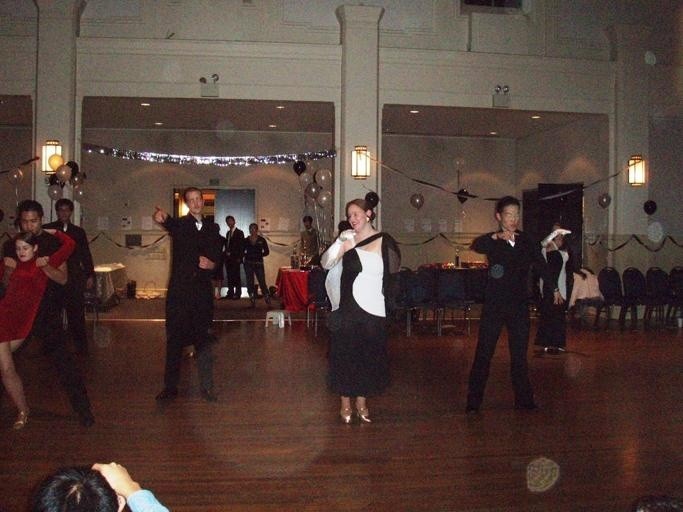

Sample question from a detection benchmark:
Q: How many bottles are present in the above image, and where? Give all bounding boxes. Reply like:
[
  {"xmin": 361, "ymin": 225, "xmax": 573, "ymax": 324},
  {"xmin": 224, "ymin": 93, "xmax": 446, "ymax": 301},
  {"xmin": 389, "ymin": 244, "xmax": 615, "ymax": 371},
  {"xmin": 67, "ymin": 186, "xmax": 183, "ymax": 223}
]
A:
[
  {"xmin": 300, "ymin": 253, "xmax": 305, "ymax": 269},
  {"xmin": 455, "ymin": 249, "xmax": 460, "ymax": 268},
  {"xmin": 290, "ymin": 248, "xmax": 299, "ymax": 269}
]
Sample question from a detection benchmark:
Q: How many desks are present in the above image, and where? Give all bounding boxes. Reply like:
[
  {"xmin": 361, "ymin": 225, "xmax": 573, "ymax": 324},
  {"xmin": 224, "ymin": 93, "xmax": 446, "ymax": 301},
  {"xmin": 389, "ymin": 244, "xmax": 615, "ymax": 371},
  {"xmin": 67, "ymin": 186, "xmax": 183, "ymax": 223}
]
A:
[
  {"xmin": 94, "ymin": 262, "xmax": 128, "ymax": 311},
  {"xmin": 276, "ymin": 265, "xmax": 316, "ymax": 328}
]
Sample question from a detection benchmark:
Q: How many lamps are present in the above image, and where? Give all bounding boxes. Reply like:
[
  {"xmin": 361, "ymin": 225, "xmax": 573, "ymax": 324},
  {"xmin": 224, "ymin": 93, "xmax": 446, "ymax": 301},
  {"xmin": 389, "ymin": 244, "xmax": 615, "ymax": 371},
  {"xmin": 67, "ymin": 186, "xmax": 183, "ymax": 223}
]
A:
[
  {"xmin": 212, "ymin": 74, "xmax": 219, "ymax": 84},
  {"xmin": 494, "ymin": 84, "xmax": 501, "ymax": 95},
  {"xmin": 628, "ymin": 155, "xmax": 646, "ymax": 186},
  {"xmin": 40, "ymin": 139, "xmax": 63, "ymax": 175},
  {"xmin": 503, "ymin": 85, "xmax": 509, "ymax": 95},
  {"xmin": 352, "ymin": 145, "xmax": 370, "ymax": 182}
]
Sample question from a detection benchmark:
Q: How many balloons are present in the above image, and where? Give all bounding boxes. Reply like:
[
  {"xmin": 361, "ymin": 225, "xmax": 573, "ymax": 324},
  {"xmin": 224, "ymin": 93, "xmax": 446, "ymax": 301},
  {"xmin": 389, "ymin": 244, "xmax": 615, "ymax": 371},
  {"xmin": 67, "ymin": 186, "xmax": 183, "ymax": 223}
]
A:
[
  {"xmin": 367, "ymin": 193, "xmax": 379, "ymax": 208},
  {"xmin": 293, "ymin": 158, "xmax": 333, "ymax": 208},
  {"xmin": 48, "ymin": 155, "xmax": 87, "ymax": 199},
  {"xmin": 599, "ymin": 193, "xmax": 611, "ymax": 209},
  {"xmin": 410, "ymin": 194, "xmax": 425, "ymax": 210},
  {"xmin": 643, "ymin": 200, "xmax": 656, "ymax": 216},
  {"xmin": 7, "ymin": 167, "xmax": 24, "ymax": 185}
]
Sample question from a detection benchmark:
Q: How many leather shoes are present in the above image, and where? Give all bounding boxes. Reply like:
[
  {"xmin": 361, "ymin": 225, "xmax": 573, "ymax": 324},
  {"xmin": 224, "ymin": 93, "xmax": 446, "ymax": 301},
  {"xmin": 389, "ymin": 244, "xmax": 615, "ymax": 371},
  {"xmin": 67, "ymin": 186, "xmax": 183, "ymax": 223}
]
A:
[
  {"xmin": 154, "ymin": 388, "xmax": 177, "ymax": 403},
  {"xmin": 201, "ymin": 388, "xmax": 218, "ymax": 403},
  {"xmin": 76, "ymin": 407, "xmax": 94, "ymax": 428},
  {"xmin": 513, "ymin": 403, "xmax": 542, "ymax": 413},
  {"xmin": 463, "ymin": 400, "xmax": 480, "ymax": 414}
]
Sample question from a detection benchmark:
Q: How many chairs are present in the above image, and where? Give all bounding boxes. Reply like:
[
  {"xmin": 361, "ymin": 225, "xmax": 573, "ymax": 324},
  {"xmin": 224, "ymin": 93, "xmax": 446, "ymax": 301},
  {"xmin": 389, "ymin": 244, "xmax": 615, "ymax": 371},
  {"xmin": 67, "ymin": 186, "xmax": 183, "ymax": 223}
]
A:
[
  {"xmin": 399, "ymin": 270, "xmax": 439, "ymax": 336},
  {"xmin": 669, "ymin": 266, "xmax": 683, "ymax": 328},
  {"xmin": 623, "ymin": 271, "xmax": 663, "ymax": 325},
  {"xmin": 596, "ymin": 269, "xmax": 631, "ymax": 327},
  {"xmin": 438, "ymin": 267, "xmax": 475, "ymax": 336},
  {"xmin": 567, "ymin": 268, "xmax": 605, "ymax": 330},
  {"xmin": 644, "ymin": 268, "xmax": 677, "ymax": 324}
]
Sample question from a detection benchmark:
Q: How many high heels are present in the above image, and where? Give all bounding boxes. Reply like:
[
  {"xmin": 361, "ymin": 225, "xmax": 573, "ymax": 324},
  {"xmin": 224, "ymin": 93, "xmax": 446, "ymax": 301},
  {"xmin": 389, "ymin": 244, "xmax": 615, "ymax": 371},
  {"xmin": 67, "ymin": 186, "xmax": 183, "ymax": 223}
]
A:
[
  {"xmin": 14, "ymin": 406, "xmax": 29, "ymax": 431},
  {"xmin": 340, "ymin": 398, "xmax": 353, "ymax": 424},
  {"xmin": 354, "ymin": 403, "xmax": 371, "ymax": 423}
]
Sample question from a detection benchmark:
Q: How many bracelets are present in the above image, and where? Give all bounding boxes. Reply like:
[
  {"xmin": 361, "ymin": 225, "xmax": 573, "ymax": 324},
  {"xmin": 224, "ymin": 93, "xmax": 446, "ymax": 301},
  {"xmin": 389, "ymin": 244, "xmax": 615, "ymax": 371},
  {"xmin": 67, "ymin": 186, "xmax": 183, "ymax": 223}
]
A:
[{"xmin": 339, "ymin": 237, "xmax": 348, "ymax": 240}]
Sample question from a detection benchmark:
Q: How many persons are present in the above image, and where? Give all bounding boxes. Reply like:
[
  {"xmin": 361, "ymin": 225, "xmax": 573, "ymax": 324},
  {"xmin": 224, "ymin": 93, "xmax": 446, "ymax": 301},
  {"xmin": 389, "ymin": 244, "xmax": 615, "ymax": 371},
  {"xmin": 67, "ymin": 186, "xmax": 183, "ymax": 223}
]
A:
[
  {"xmin": 319, "ymin": 198, "xmax": 402, "ymax": 425},
  {"xmin": 1, "ymin": 200, "xmax": 94, "ymax": 427},
  {"xmin": 0, "ymin": 229, "xmax": 76, "ymax": 431},
  {"xmin": 457, "ymin": 190, "xmax": 581, "ymax": 411},
  {"xmin": 208, "ymin": 215, "xmax": 272, "ymax": 308},
  {"xmin": 299, "ymin": 216, "xmax": 319, "ymax": 265},
  {"xmin": 41, "ymin": 198, "xmax": 96, "ymax": 359},
  {"xmin": 30, "ymin": 461, "xmax": 169, "ymax": 511},
  {"xmin": 153, "ymin": 189, "xmax": 223, "ymax": 403}
]
